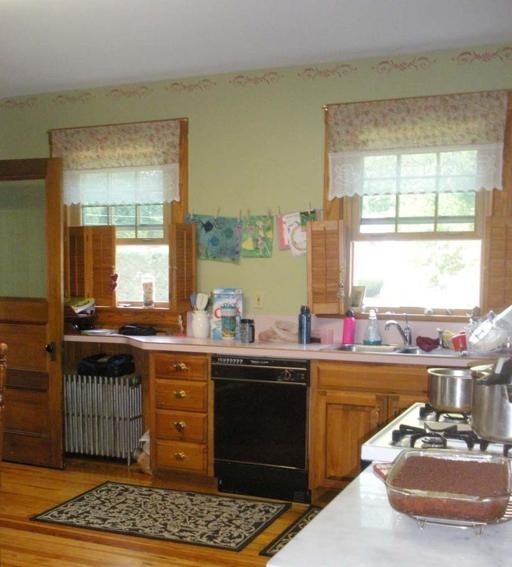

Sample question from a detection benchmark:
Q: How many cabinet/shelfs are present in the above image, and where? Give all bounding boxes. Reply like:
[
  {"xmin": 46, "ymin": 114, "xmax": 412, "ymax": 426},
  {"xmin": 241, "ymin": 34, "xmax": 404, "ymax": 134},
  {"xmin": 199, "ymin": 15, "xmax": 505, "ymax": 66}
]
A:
[
  {"xmin": 309, "ymin": 360, "xmax": 428, "ymax": 509},
  {"xmin": 147, "ymin": 352, "xmax": 214, "ymax": 491}
]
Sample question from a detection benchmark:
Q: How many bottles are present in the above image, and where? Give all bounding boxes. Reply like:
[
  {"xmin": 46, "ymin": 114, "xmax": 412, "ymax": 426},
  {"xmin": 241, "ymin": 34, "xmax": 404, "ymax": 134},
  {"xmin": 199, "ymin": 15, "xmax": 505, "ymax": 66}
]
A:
[
  {"xmin": 342, "ymin": 309, "xmax": 355, "ymax": 344},
  {"xmin": 192, "ymin": 310, "xmax": 210, "ymax": 340},
  {"xmin": 239, "ymin": 319, "xmax": 255, "ymax": 346},
  {"xmin": 220, "ymin": 306, "xmax": 237, "ymax": 340},
  {"xmin": 142, "ymin": 275, "xmax": 154, "ymax": 308},
  {"xmin": 299, "ymin": 305, "xmax": 311, "ymax": 345}
]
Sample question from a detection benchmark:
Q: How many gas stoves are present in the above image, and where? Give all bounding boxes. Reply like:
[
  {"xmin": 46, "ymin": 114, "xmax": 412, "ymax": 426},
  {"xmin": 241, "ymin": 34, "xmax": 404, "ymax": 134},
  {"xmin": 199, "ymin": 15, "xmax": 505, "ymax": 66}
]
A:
[{"xmin": 361, "ymin": 402, "xmax": 511, "ymax": 462}]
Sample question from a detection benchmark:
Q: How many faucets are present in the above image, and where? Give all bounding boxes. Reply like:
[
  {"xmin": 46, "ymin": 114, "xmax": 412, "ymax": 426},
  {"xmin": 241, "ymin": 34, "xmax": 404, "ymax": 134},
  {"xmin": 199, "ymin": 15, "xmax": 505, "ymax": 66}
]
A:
[{"xmin": 384, "ymin": 314, "xmax": 412, "ymax": 347}]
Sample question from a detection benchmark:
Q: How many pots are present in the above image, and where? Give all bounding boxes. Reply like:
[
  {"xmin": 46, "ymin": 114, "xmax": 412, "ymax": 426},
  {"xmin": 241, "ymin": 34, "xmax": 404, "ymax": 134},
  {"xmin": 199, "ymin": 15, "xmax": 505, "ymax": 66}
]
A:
[
  {"xmin": 426, "ymin": 367, "xmax": 472, "ymax": 414},
  {"xmin": 470, "ymin": 358, "xmax": 510, "ymax": 445}
]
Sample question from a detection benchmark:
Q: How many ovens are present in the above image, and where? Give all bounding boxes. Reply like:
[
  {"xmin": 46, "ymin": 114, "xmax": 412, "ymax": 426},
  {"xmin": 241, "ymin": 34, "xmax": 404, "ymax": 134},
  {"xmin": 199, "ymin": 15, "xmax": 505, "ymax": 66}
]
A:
[{"xmin": 209, "ymin": 354, "xmax": 311, "ymax": 506}]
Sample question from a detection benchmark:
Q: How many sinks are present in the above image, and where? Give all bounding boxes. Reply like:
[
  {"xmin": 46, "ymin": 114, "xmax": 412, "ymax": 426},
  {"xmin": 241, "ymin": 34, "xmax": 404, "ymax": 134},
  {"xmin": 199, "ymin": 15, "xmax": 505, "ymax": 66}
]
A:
[
  {"xmin": 322, "ymin": 344, "xmax": 400, "ymax": 355},
  {"xmin": 398, "ymin": 345, "xmax": 464, "ymax": 357}
]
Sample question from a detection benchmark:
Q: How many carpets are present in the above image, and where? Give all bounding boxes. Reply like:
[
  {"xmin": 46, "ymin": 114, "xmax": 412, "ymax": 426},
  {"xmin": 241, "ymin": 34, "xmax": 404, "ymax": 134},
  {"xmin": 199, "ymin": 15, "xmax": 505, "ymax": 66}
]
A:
[{"xmin": 29, "ymin": 480, "xmax": 321, "ymax": 558}]
